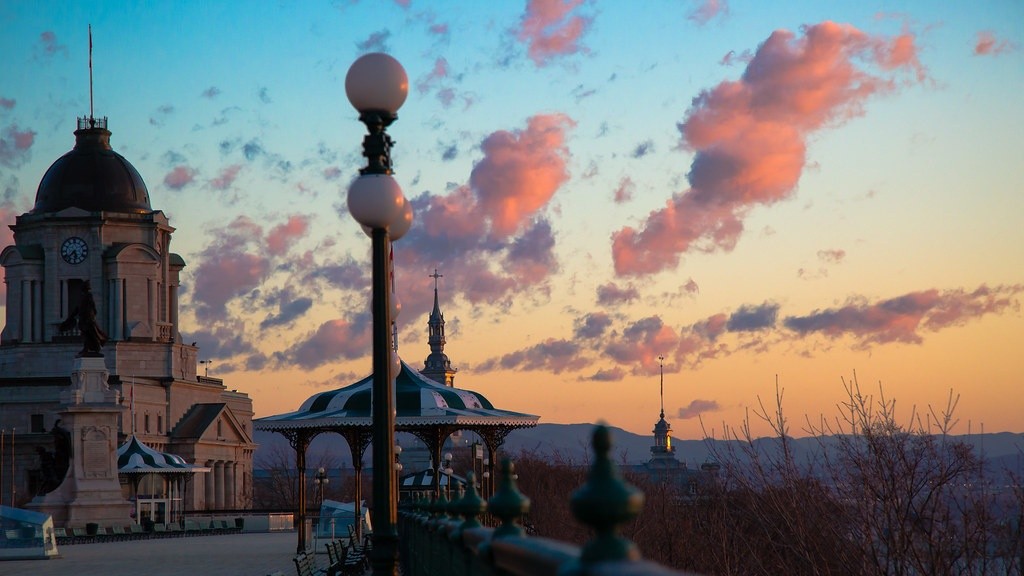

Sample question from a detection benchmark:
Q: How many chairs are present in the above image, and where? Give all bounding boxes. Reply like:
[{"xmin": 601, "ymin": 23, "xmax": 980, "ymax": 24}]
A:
[{"xmin": 292, "ymin": 523, "xmax": 370, "ymax": 576}]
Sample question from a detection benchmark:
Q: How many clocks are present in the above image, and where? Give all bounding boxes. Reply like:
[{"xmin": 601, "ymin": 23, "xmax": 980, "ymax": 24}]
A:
[{"xmin": 61, "ymin": 236, "xmax": 88, "ymax": 265}]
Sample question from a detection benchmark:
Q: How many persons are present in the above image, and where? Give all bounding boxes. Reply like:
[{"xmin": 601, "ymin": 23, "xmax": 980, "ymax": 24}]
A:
[{"xmin": 72, "ymin": 279, "xmax": 111, "ymax": 355}]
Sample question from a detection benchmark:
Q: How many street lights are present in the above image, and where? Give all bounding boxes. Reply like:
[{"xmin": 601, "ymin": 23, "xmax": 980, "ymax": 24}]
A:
[{"xmin": 346, "ymin": 50, "xmax": 410, "ymax": 576}]
